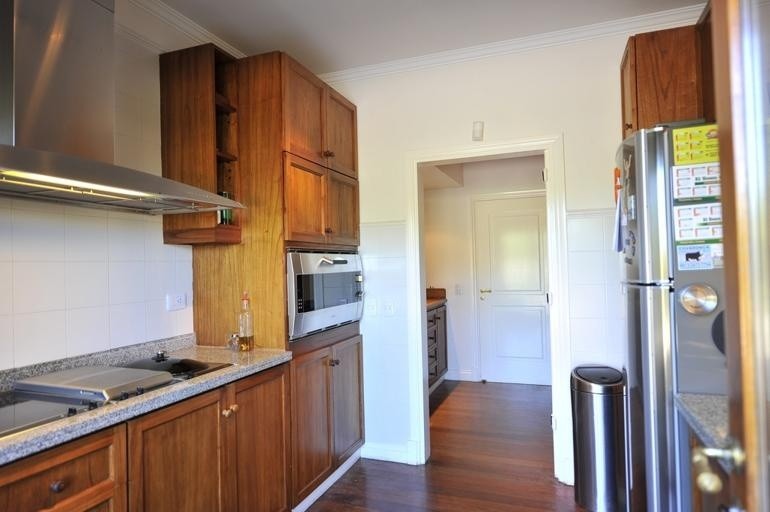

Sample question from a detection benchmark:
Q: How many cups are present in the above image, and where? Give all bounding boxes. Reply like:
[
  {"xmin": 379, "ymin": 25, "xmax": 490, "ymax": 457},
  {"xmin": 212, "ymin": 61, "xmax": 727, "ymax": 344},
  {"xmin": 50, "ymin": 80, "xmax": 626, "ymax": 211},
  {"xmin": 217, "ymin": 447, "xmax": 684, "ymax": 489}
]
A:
[{"xmin": 227, "ymin": 330, "xmax": 240, "ymax": 354}]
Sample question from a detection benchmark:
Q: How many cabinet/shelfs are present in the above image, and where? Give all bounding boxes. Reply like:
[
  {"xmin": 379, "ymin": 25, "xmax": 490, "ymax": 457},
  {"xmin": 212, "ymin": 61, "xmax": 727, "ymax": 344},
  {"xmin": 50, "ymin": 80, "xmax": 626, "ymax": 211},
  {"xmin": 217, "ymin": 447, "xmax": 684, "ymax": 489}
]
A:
[
  {"xmin": 192, "ymin": 49, "xmax": 359, "ymax": 351},
  {"xmin": 0, "ymin": 403, "xmax": 127, "ymax": 512},
  {"xmin": 159, "ymin": 42, "xmax": 241, "ymax": 245},
  {"xmin": 619, "ymin": 23, "xmax": 702, "ymax": 140},
  {"xmin": 427, "ymin": 305, "xmax": 448, "ymax": 397},
  {"xmin": 128, "ymin": 353, "xmax": 291, "ymax": 511},
  {"xmin": 292, "ymin": 334, "xmax": 366, "ymax": 511}
]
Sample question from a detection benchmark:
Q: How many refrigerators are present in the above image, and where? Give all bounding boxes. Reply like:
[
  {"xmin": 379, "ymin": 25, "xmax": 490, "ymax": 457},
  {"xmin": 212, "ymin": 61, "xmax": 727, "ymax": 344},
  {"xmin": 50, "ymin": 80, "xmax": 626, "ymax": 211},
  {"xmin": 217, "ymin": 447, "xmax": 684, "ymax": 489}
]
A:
[{"xmin": 613, "ymin": 120, "xmax": 725, "ymax": 510}]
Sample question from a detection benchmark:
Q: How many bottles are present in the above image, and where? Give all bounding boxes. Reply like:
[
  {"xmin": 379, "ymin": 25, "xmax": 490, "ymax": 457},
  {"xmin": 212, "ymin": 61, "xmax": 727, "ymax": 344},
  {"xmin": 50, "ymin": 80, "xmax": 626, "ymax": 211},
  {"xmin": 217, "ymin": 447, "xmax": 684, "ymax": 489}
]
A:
[{"xmin": 238, "ymin": 292, "xmax": 254, "ymax": 352}]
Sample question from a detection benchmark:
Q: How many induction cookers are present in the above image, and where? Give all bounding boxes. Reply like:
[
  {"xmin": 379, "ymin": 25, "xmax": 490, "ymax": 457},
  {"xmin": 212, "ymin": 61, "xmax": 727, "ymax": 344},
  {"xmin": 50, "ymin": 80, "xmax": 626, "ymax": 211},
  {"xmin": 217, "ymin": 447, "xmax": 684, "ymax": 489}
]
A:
[{"xmin": 1, "ymin": 363, "xmax": 236, "ymax": 435}]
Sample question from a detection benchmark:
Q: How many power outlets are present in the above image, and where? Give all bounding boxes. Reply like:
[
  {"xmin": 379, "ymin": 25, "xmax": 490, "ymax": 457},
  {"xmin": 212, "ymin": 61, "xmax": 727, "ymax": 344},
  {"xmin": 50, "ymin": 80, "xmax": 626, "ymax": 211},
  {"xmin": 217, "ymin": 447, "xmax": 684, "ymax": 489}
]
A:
[{"xmin": 167, "ymin": 292, "xmax": 186, "ymax": 312}]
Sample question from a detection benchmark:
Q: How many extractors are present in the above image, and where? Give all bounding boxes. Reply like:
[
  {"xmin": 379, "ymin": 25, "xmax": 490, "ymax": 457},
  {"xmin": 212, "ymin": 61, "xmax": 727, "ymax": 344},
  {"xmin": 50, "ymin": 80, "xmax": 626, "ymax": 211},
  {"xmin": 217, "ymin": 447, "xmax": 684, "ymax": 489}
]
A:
[{"xmin": 0, "ymin": 139, "xmax": 245, "ymax": 216}]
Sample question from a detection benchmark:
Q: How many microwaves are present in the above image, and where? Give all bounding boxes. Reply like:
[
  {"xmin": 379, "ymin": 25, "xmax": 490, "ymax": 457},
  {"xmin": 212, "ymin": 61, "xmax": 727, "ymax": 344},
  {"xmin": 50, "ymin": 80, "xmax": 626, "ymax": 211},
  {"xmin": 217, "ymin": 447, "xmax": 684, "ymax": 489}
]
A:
[{"xmin": 286, "ymin": 251, "xmax": 365, "ymax": 342}]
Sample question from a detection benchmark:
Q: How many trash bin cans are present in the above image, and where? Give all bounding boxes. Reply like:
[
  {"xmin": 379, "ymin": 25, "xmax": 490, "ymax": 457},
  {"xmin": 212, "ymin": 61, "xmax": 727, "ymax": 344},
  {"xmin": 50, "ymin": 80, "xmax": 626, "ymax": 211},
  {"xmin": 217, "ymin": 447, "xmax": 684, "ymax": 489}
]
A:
[{"xmin": 570, "ymin": 364, "xmax": 627, "ymax": 512}]
